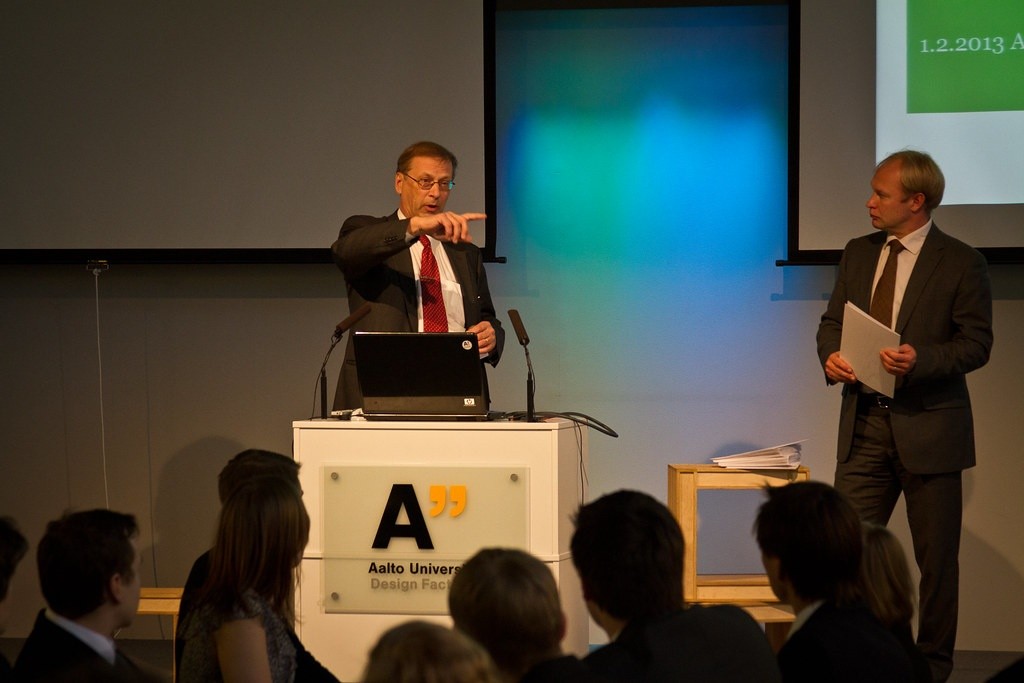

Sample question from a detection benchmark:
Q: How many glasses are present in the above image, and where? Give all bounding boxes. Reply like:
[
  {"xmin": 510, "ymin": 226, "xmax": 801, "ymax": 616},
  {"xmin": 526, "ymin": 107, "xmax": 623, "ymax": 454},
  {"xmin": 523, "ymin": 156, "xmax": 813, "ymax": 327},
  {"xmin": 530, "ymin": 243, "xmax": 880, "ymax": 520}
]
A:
[{"xmin": 404, "ymin": 171, "xmax": 455, "ymax": 191}]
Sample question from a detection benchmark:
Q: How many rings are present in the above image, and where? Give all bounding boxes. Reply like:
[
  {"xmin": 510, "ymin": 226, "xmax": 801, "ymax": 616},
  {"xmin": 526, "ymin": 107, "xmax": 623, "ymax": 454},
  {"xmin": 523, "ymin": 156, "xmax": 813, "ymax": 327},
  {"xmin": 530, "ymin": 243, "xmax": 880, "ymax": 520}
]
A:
[
  {"xmin": 894, "ymin": 366, "xmax": 897, "ymax": 372},
  {"xmin": 485, "ymin": 339, "xmax": 489, "ymax": 345}
]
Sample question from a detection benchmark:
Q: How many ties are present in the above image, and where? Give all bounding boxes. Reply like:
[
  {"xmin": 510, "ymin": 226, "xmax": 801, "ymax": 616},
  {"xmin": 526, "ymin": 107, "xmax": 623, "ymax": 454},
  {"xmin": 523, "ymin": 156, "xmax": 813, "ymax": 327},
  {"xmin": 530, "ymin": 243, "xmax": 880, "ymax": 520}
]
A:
[
  {"xmin": 419, "ymin": 235, "xmax": 448, "ymax": 333},
  {"xmin": 869, "ymin": 240, "xmax": 907, "ymax": 329}
]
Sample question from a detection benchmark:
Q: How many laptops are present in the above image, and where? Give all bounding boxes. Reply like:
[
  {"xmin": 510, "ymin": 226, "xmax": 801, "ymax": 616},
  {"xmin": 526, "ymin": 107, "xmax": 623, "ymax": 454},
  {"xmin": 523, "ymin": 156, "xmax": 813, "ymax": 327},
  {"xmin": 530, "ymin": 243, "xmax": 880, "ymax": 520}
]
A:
[{"xmin": 351, "ymin": 332, "xmax": 506, "ymax": 422}]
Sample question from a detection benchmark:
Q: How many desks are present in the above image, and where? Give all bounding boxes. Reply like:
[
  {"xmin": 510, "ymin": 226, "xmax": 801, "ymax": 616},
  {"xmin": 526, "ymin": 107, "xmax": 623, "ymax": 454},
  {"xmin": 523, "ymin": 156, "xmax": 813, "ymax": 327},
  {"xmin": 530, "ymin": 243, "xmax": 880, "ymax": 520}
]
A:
[{"xmin": 667, "ymin": 464, "xmax": 809, "ymax": 608}]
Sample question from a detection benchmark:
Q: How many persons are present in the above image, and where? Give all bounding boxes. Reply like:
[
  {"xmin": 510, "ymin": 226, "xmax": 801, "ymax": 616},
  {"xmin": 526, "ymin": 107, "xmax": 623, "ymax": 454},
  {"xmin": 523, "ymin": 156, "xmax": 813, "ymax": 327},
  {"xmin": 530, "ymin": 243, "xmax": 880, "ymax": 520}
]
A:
[
  {"xmin": 448, "ymin": 546, "xmax": 601, "ymax": 682},
  {"xmin": 330, "ymin": 141, "xmax": 507, "ymax": 421},
  {"xmin": 175, "ymin": 448, "xmax": 342, "ymax": 682},
  {"xmin": 12, "ymin": 508, "xmax": 151, "ymax": 683},
  {"xmin": 177, "ymin": 476, "xmax": 312, "ymax": 683},
  {"xmin": 359, "ymin": 621, "xmax": 512, "ymax": 682},
  {"xmin": 570, "ymin": 488, "xmax": 785, "ymax": 683},
  {"xmin": 750, "ymin": 478, "xmax": 931, "ymax": 682},
  {"xmin": 0, "ymin": 516, "xmax": 29, "ymax": 683},
  {"xmin": 816, "ymin": 150, "xmax": 994, "ymax": 683}
]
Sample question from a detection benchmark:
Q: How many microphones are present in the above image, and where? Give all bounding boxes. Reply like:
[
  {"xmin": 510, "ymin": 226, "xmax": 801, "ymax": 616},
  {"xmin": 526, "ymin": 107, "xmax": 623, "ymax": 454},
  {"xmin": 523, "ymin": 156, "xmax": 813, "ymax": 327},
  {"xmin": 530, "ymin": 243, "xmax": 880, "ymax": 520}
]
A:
[
  {"xmin": 333, "ymin": 302, "xmax": 372, "ymax": 338},
  {"xmin": 507, "ymin": 309, "xmax": 530, "ymax": 347}
]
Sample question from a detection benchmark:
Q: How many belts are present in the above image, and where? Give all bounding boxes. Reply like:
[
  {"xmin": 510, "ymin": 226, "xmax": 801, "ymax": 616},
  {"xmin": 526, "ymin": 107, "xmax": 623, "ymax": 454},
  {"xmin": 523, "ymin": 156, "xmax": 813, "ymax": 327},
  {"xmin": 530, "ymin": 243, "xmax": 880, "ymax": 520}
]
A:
[{"xmin": 868, "ymin": 395, "xmax": 889, "ymax": 408}]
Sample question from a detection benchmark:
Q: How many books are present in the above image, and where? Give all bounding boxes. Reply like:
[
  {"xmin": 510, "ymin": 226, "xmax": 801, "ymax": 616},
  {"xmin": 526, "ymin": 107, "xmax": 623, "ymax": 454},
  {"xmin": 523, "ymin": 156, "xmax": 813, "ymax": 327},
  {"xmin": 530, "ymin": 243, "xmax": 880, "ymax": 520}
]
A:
[
  {"xmin": 837, "ymin": 300, "xmax": 901, "ymax": 398},
  {"xmin": 708, "ymin": 438, "xmax": 809, "ymax": 470}
]
